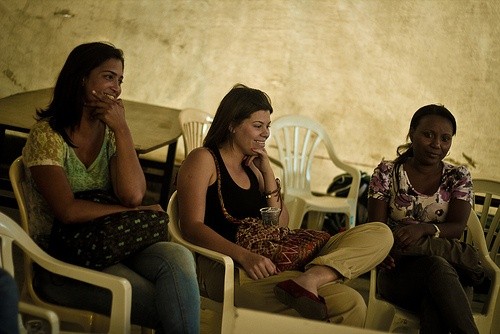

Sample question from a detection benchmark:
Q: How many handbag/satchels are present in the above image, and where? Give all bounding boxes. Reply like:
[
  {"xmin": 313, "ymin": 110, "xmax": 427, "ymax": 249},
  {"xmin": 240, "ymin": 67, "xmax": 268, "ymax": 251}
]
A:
[
  {"xmin": 51, "ymin": 188, "xmax": 170, "ymax": 268},
  {"xmin": 390, "ymin": 235, "xmax": 491, "ymax": 295},
  {"xmin": 236, "ymin": 217, "xmax": 331, "ymax": 270}
]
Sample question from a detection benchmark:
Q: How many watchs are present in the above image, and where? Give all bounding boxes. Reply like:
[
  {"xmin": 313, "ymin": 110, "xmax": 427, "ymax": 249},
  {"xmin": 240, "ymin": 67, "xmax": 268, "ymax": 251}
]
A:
[{"xmin": 432, "ymin": 224, "xmax": 441, "ymax": 239}]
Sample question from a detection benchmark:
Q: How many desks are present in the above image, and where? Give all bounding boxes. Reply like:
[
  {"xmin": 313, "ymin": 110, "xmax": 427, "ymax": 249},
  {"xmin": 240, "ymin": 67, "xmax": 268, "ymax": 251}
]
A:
[{"xmin": 0, "ymin": 88, "xmax": 183, "ymax": 213}]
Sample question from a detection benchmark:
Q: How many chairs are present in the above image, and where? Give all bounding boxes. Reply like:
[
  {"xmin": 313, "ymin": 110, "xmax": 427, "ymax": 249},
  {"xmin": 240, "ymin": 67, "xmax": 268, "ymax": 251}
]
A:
[{"xmin": 0, "ymin": 109, "xmax": 500, "ymax": 334}]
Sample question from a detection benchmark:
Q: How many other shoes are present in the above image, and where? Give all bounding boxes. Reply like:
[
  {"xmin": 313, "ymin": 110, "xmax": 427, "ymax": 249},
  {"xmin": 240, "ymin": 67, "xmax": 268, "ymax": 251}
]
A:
[{"xmin": 275, "ymin": 279, "xmax": 328, "ymax": 321}]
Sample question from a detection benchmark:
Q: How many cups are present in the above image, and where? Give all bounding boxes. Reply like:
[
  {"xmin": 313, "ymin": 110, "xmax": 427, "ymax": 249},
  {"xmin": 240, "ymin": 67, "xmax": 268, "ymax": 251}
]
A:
[{"xmin": 260, "ymin": 207, "xmax": 281, "ymax": 225}]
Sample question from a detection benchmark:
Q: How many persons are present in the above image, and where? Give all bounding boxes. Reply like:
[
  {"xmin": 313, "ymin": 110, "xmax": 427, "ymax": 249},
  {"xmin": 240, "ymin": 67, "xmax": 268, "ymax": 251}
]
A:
[
  {"xmin": 23, "ymin": 43, "xmax": 201, "ymax": 334},
  {"xmin": 177, "ymin": 83, "xmax": 394, "ymax": 329},
  {"xmin": 368, "ymin": 105, "xmax": 480, "ymax": 334}
]
